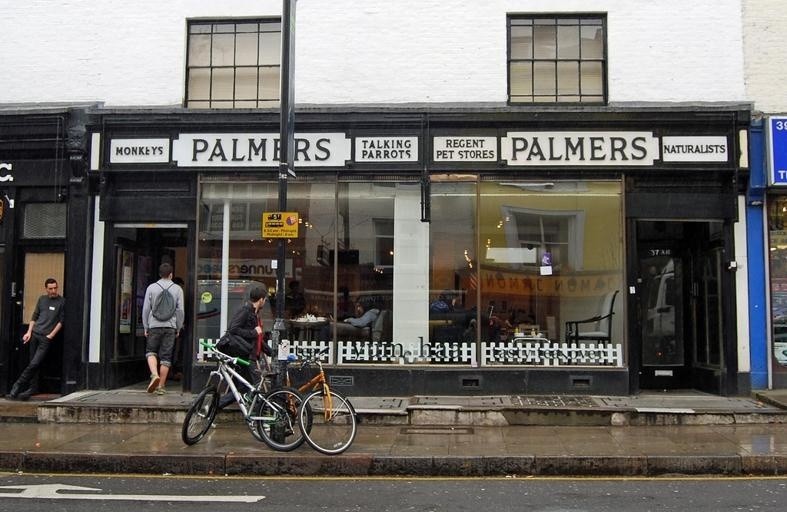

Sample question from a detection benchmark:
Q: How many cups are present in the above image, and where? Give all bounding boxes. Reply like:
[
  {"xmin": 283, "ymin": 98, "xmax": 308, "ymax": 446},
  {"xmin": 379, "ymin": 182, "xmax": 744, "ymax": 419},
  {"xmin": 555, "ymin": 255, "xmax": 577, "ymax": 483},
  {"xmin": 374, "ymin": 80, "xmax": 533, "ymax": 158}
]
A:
[{"xmin": 296, "ymin": 315, "xmax": 327, "ymax": 323}]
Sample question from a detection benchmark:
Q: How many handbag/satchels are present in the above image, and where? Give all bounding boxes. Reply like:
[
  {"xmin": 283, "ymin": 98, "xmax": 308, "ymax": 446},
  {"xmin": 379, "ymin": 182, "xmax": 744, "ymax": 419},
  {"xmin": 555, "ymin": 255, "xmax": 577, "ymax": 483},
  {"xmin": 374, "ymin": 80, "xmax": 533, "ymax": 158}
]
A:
[{"xmin": 215, "ymin": 329, "xmax": 253, "ymax": 358}]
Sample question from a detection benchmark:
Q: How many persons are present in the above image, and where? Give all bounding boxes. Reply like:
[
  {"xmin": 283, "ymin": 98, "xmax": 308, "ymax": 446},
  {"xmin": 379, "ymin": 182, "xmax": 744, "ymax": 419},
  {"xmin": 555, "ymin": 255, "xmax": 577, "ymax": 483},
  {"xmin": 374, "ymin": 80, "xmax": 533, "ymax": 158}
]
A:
[
  {"xmin": 431, "ymin": 288, "xmax": 458, "ymax": 314},
  {"xmin": 3, "ymin": 278, "xmax": 66, "ymax": 402},
  {"xmin": 283, "ymin": 278, "xmax": 307, "ymax": 342},
  {"xmin": 342, "ymin": 294, "xmax": 380, "ymax": 336},
  {"xmin": 194, "ymin": 286, "xmax": 278, "ymax": 428},
  {"xmin": 326, "ymin": 286, "xmax": 353, "ymax": 322},
  {"xmin": 166, "ymin": 277, "xmax": 187, "ymax": 383},
  {"xmin": 141, "ymin": 262, "xmax": 185, "ymax": 392}
]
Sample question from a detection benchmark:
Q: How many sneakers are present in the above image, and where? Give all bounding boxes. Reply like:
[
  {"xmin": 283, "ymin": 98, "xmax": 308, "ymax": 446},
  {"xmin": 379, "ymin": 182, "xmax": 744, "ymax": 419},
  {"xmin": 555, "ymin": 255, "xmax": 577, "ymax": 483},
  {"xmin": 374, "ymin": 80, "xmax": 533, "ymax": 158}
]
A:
[
  {"xmin": 157, "ymin": 386, "xmax": 168, "ymax": 396},
  {"xmin": 262, "ymin": 422, "xmax": 271, "ymax": 432},
  {"xmin": 146, "ymin": 376, "xmax": 161, "ymax": 393},
  {"xmin": 17, "ymin": 388, "xmax": 39, "ymax": 402},
  {"xmin": 7, "ymin": 384, "xmax": 21, "ymax": 401},
  {"xmin": 205, "ymin": 404, "xmax": 217, "ymax": 429}
]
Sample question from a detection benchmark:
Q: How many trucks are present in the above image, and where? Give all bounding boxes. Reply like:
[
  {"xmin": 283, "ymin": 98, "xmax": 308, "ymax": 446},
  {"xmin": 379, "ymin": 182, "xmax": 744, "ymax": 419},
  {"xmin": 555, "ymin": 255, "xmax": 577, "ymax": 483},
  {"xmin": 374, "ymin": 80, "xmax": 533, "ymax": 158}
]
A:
[{"xmin": 641, "ymin": 255, "xmax": 679, "ymax": 364}]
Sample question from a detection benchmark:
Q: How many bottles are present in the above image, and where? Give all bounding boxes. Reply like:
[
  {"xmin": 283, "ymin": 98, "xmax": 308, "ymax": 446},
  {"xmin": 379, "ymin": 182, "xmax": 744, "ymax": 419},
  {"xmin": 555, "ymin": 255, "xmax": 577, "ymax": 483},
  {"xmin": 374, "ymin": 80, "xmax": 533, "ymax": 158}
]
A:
[
  {"xmin": 244, "ymin": 388, "xmax": 256, "ymax": 407},
  {"xmin": 514, "ymin": 327, "xmax": 535, "ymax": 338}
]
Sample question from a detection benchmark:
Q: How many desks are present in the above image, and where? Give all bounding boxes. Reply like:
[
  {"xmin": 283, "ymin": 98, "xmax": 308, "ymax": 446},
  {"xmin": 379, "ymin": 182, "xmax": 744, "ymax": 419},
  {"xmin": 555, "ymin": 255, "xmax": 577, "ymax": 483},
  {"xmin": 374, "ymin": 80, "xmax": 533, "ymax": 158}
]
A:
[{"xmin": 283, "ymin": 315, "xmax": 328, "ymax": 340}]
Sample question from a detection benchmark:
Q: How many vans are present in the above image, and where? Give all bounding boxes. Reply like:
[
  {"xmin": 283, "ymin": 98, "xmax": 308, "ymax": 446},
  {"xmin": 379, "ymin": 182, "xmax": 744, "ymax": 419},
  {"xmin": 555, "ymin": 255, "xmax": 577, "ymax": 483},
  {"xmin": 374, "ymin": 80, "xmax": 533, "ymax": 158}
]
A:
[{"xmin": 193, "ymin": 277, "xmax": 274, "ymax": 338}]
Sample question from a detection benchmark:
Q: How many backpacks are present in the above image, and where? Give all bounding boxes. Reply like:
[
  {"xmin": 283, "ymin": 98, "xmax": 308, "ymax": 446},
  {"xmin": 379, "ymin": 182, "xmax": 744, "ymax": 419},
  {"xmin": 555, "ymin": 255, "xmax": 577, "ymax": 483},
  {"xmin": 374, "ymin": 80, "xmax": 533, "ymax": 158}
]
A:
[{"xmin": 150, "ymin": 282, "xmax": 177, "ymax": 323}]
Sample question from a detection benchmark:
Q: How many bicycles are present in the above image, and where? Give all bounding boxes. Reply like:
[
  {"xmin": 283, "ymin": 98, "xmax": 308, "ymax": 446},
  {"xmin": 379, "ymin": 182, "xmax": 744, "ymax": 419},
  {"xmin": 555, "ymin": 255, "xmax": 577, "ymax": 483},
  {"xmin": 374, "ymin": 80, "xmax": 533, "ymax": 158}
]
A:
[
  {"xmin": 181, "ymin": 337, "xmax": 313, "ymax": 452},
  {"xmin": 238, "ymin": 346, "xmax": 358, "ymax": 457}
]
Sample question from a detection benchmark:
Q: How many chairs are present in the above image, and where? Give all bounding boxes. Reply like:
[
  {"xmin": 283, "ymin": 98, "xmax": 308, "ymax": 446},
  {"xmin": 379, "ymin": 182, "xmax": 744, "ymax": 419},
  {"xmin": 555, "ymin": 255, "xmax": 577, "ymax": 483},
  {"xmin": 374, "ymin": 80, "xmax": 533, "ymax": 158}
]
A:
[
  {"xmin": 565, "ymin": 288, "xmax": 619, "ymax": 366},
  {"xmin": 329, "ymin": 306, "xmax": 391, "ymax": 343}
]
